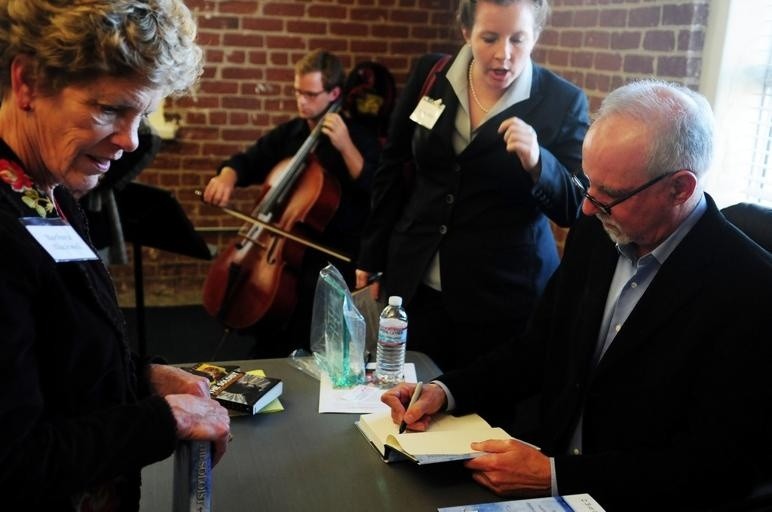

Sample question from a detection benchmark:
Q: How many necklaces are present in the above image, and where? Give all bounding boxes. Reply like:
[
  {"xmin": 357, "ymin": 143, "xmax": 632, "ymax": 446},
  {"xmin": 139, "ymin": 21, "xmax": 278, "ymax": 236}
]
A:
[{"xmin": 468, "ymin": 59, "xmax": 491, "ymax": 113}]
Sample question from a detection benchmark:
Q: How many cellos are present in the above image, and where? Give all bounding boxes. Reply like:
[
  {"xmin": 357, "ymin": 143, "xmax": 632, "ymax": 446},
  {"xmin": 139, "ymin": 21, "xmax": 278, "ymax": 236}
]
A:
[{"xmin": 201, "ymin": 65, "xmax": 375, "ymax": 329}]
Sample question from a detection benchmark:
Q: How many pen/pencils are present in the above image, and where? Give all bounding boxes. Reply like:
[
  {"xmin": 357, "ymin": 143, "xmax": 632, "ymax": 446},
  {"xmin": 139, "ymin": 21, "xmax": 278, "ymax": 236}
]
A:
[
  {"xmin": 368, "ymin": 271, "xmax": 384, "ymax": 284},
  {"xmin": 399, "ymin": 381, "xmax": 423, "ymax": 433}
]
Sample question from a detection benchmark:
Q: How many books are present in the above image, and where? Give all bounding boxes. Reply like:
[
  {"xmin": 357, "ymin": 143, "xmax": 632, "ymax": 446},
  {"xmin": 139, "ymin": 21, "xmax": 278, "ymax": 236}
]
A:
[
  {"xmin": 355, "ymin": 409, "xmax": 542, "ymax": 467},
  {"xmin": 185, "ymin": 361, "xmax": 286, "ymax": 416},
  {"xmin": 225, "ymin": 367, "xmax": 286, "ymax": 417},
  {"xmin": 436, "ymin": 491, "xmax": 606, "ymax": 510}
]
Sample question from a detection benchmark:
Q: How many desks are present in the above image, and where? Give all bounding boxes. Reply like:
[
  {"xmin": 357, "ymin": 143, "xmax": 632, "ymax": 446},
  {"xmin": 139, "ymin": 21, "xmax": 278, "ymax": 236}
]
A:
[{"xmin": 140, "ymin": 352, "xmax": 585, "ymax": 512}]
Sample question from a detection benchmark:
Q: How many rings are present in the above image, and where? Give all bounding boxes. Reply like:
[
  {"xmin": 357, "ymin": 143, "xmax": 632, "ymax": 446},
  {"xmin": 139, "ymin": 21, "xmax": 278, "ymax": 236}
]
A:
[{"xmin": 228, "ymin": 432, "xmax": 233, "ymax": 442}]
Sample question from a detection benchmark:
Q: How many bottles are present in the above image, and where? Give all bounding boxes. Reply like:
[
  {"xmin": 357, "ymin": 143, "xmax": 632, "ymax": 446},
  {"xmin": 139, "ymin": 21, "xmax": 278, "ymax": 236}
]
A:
[{"xmin": 373, "ymin": 294, "xmax": 408, "ymax": 386}]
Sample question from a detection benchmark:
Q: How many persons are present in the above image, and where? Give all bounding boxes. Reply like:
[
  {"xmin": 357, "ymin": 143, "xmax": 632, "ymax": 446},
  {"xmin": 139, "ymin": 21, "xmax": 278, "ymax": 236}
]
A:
[
  {"xmin": 354, "ymin": 0, "xmax": 591, "ymax": 374},
  {"xmin": 202, "ymin": 49, "xmax": 381, "ymax": 356},
  {"xmin": 379, "ymin": 80, "xmax": 769, "ymax": 511},
  {"xmin": 0, "ymin": 1, "xmax": 237, "ymax": 511}
]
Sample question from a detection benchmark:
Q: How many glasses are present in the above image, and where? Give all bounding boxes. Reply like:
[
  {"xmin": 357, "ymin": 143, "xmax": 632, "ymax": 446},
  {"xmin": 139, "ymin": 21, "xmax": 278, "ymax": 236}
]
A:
[
  {"xmin": 293, "ymin": 89, "xmax": 326, "ymax": 99},
  {"xmin": 571, "ymin": 168, "xmax": 668, "ymax": 216}
]
